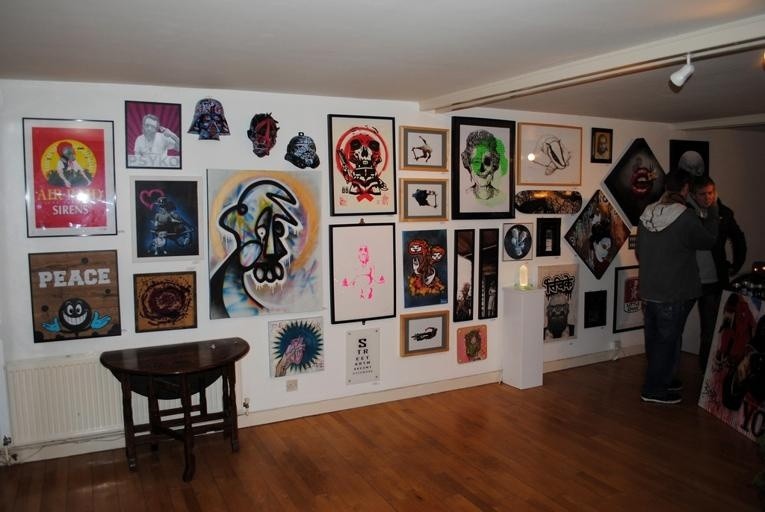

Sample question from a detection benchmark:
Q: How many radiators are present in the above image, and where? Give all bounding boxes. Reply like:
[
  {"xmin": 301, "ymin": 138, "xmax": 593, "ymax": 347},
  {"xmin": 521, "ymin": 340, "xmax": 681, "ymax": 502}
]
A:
[{"xmin": 0, "ymin": 349, "xmax": 251, "ymax": 452}]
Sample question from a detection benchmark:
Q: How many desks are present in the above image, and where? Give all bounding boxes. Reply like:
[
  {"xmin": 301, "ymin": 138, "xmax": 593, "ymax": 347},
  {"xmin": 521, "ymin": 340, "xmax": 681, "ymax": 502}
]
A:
[{"xmin": 98, "ymin": 337, "xmax": 253, "ymax": 484}]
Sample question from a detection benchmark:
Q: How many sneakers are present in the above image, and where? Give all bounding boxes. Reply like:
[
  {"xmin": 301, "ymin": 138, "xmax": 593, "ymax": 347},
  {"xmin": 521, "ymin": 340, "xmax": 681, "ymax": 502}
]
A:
[
  {"xmin": 666, "ymin": 379, "xmax": 682, "ymax": 391},
  {"xmin": 640, "ymin": 387, "xmax": 682, "ymax": 405}
]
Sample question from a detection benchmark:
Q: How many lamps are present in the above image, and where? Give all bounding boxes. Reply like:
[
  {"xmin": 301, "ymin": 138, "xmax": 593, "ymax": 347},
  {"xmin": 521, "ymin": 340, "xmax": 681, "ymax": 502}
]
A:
[{"xmin": 665, "ymin": 53, "xmax": 697, "ymax": 89}]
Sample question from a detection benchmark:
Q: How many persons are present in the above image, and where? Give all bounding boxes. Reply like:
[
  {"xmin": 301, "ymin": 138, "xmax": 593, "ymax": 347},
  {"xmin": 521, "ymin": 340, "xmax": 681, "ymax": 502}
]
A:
[
  {"xmin": 461, "ymin": 129, "xmax": 509, "ymax": 207},
  {"xmin": 543, "ymin": 295, "xmax": 560, "ymax": 340},
  {"xmin": 586, "ymin": 220, "xmax": 611, "ymax": 263},
  {"xmin": 487, "ymin": 279, "xmax": 498, "ymax": 317},
  {"xmin": 560, "ymin": 295, "xmax": 574, "ymax": 338},
  {"xmin": 132, "ymin": 113, "xmax": 181, "ymax": 164},
  {"xmin": 635, "ymin": 167, "xmax": 713, "ymax": 404},
  {"xmin": 55, "ymin": 147, "xmax": 93, "ymax": 189},
  {"xmin": 682, "ymin": 177, "xmax": 746, "ymax": 376}
]
